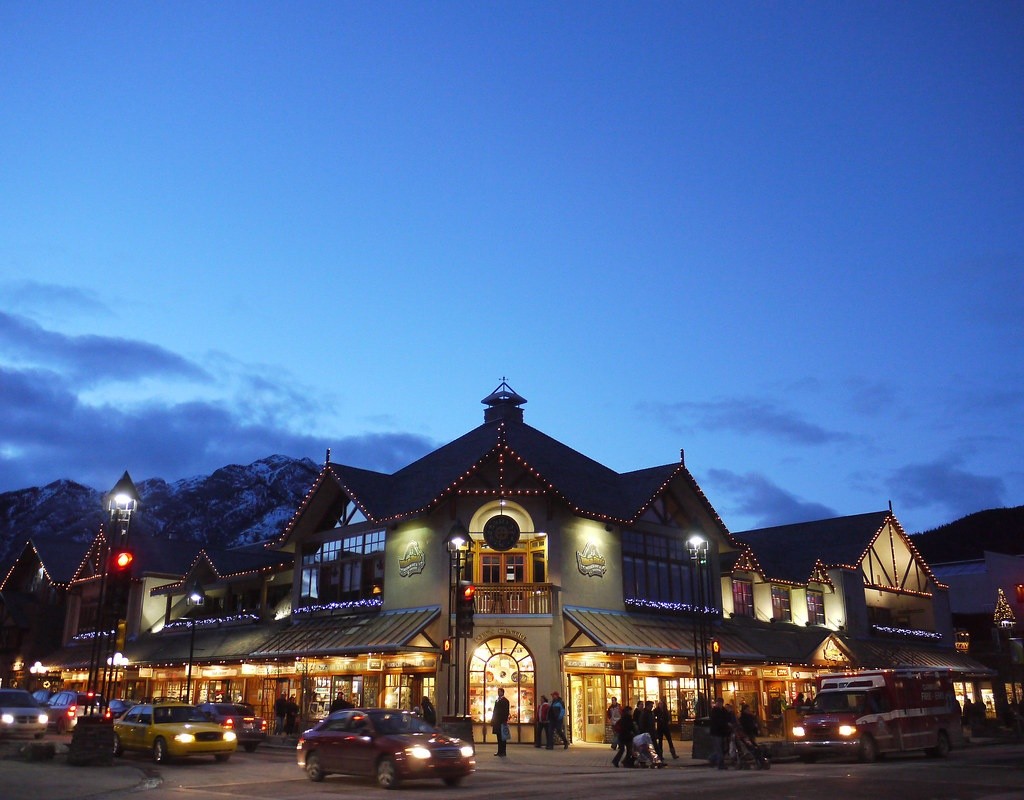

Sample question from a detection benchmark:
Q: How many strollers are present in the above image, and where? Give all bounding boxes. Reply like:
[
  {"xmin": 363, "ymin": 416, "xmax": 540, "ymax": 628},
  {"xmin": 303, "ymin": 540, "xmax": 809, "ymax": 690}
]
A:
[
  {"xmin": 726, "ymin": 722, "xmax": 771, "ymax": 770},
  {"xmin": 633, "ymin": 732, "xmax": 672, "ymax": 768}
]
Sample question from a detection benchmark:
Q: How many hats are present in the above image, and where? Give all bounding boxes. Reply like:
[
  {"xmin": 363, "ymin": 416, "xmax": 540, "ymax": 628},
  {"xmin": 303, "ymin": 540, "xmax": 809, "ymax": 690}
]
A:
[
  {"xmin": 715, "ymin": 698, "xmax": 724, "ymax": 703},
  {"xmin": 551, "ymin": 692, "xmax": 559, "ymax": 697}
]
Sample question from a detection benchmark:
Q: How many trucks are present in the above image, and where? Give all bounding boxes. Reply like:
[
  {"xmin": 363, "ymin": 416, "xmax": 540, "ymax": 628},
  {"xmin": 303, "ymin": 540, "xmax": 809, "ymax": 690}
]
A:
[{"xmin": 791, "ymin": 667, "xmax": 965, "ymax": 764}]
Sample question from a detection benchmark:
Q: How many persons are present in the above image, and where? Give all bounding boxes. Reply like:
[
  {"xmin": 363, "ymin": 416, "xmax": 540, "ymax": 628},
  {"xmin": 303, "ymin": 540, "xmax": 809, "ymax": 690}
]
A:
[
  {"xmin": 707, "ymin": 697, "xmax": 761, "ymax": 771},
  {"xmin": 489, "ymin": 687, "xmax": 510, "ymax": 756},
  {"xmin": 1009, "ymin": 698, "xmax": 1024, "ymax": 723},
  {"xmin": 140, "ymin": 697, "xmax": 147, "ymax": 705},
  {"xmin": 286, "ymin": 697, "xmax": 299, "ymax": 737},
  {"xmin": 329, "ymin": 691, "xmax": 354, "ymax": 714},
  {"xmin": 790, "ymin": 693, "xmax": 812, "ymax": 708},
  {"xmin": 545, "ymin": 691, "xmax": 570, "ymax": 750},
  {"xmin": 951, "ymin": 696, "xmax": 985, "ymax": 742},
  {"xmin": 272, "ymin": 693, "xmax": 287, "ymax": 736},
  {"xmin": 607, "ymin": 697, "xmax": 681, "ymax": 770},
  {"xmin": 534, "ymin": 695, "xmax": 551, "ymax": 748},
  {"xmin": 421, "ymin": 696, "xmax": 437, "ymax": 726}
]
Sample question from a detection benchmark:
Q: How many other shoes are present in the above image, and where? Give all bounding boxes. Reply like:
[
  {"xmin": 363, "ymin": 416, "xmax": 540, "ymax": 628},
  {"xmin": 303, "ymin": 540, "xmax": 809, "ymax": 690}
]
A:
[
  {"xmin": 564, "ymin": 742, "xmax": 570, "ymax": 749},
  {"xmin": 545, "ymin": 746, "xmax": 553, "ymax": 750},
  {"xmin": 611, "ymin": 745, "xmax": 616, "ymax": 750},
  {"xmin": 672, "ymin": 754, "xmax": 679, "ymax": 760},
  {"xmin": 612, "ymin": 759, "xmax": 619, "ymax": 768}
]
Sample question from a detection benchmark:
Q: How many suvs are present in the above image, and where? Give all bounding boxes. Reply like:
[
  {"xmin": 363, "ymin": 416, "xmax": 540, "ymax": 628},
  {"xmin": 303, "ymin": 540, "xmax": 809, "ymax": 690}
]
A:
[
  {"xmin": 46, "ymin": 691, "xmax": 111, "ymax": 734},
  {"xmin": 0, "ymin": 690, "xmax": 49, "ymax": 738}
]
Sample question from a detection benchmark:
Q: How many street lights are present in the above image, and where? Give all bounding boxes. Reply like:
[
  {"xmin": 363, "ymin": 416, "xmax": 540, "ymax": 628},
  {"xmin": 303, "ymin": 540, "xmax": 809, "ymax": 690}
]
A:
[
  {"xmin": 443, "ymin": 518, "xmax": 474, "ymax": 718},
  {"xmin": 106, "ymin": 653, "xmax": 133, "ymax": 701},
  {"xmin": 84, "ymin": 470, "xmax": 144, "ymax": 714},
  {"xmin": 684, "ymin": 535, "xmax": 726, "ymax": 720},
  {"xmin": 185, "ymin": 579, "xmax": 207, "ymax": 704},
  {"xmin": 29, "ymin": 661, "xmax": 46, "ymax": 690}
]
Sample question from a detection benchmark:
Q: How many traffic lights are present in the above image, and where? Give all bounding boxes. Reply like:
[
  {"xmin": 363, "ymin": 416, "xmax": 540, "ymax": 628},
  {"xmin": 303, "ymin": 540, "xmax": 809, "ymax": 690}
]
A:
[
  {"xmin": 460, "ymin": 585, "xmax": 474, "ymax": 622},
  {"xmin": 113, "ymin": 550, "xmax": 134, "ymax": 575},
  {"xmin": 712, "ymin": 641, "xmax": 721, "ymax": 666}
]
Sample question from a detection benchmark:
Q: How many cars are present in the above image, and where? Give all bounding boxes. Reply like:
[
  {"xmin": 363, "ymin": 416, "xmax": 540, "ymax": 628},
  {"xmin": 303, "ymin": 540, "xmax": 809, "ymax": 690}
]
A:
[
  {"xmin": 112, "ymin": 702, "xmax": 238, "ymax": 765},
  {"xmin": 109, "ymin": 699, "xmax": 129, "ymax": 718},
  {"xmin": 197, "ymin": 703, "xmax": 272, "ymax": 752},
  {"xmin": 33, "ymin": 690, "xmax": 53, "ymax": 704},
  {"xmin": 295, "ymin": 707, "xmax": 476, "ymax": 790}
]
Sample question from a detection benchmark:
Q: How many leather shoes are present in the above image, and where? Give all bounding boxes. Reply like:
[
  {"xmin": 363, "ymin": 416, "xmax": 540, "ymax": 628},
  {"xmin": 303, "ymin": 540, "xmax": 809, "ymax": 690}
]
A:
[{"xmin": 494, "ymin": 752, "xmax": 506, "ymax": 757}]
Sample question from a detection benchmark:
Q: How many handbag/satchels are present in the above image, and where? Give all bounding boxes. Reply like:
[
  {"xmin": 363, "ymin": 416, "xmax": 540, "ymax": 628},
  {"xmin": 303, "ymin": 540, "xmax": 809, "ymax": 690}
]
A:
[
  {"xmin": 501, "ymin": 724, "xmax": 511, "ymax": 741},
  {"xmin": 614, "ymin": 719, "xmax": 623, "ymax": 733}
]
